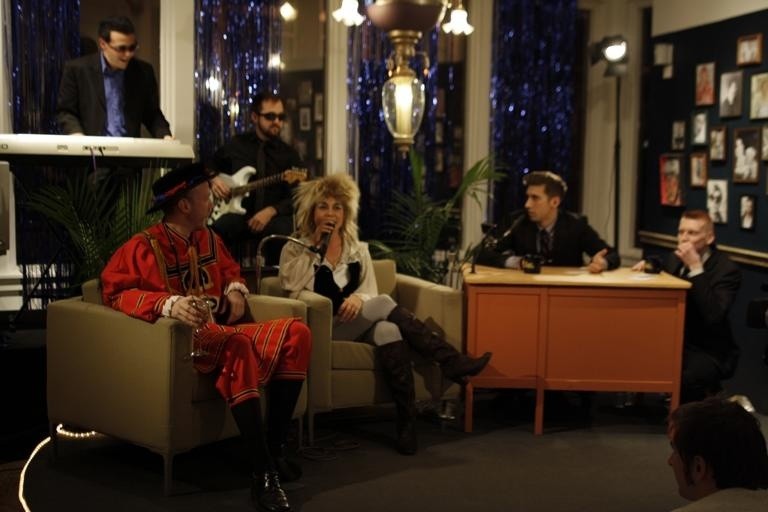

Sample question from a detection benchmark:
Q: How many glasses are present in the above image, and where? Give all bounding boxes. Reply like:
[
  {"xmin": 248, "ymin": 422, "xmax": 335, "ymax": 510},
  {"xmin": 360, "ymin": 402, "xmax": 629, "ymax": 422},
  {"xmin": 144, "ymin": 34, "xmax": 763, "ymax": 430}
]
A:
[
  {"xmin": 104, "ymin": 41, "xmax": 138, "ymax": 53},
  {"xmin": 258, "ymin": 113, "xmax": 287, "ymax": 120}
]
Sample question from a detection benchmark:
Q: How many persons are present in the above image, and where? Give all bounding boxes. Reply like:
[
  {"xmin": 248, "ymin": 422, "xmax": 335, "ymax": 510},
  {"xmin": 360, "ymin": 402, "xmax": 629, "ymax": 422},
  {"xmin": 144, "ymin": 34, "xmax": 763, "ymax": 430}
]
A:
[
  {"xmin": 94, "ymin": 158, "xmax": 317, "ymax": 512},
  {"xmin": 659, "ymin": 36, "xmax": 768, "ymax": 228},
  {"xmin": 51, "ymin": 11, "xmax": 184, "ymax": 261},
  {"xmin": 628, "ymin": 205, "xmax": 744, "ymax": 411},
  {"xmin": 278, "ymin": 171, "xmax": 494, "ymax": 458},
  {"xmin": 468, "ymin": 169, "xmax": 625, "ymax": 277},
  {"xmin": 207, "ymin": 90, "xmax": 312, "ymax": 266},
  {"xmin": 660, "ymin": 390, "xmax": 768, "ymax": 511}
]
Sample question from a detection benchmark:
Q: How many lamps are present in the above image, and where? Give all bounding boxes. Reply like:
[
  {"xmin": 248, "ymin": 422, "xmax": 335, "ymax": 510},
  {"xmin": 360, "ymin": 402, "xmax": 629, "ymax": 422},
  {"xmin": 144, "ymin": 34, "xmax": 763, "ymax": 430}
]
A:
[
  {"xmin": 326, "ymin": 1, "xmax": 486, "ymax": 161},
  {"xmin": 588, "ymin": 35, "xmax": 630, "ymax": 250}
]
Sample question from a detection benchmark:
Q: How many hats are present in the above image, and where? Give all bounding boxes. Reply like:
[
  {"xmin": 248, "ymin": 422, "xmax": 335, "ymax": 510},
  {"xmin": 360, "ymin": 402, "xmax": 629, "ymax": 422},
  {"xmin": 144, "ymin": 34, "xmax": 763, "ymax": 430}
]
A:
[{"xmin": 146, "ymin": 163, "xmax": 219, "ymax": 215}]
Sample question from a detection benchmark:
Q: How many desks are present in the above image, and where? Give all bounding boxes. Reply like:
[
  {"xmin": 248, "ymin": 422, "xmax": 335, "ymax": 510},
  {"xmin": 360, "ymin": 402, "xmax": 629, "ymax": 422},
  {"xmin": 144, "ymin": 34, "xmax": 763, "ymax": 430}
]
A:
[{"xmin": 464, "ymin": 259, "xmax": 692, "ymax": 448}]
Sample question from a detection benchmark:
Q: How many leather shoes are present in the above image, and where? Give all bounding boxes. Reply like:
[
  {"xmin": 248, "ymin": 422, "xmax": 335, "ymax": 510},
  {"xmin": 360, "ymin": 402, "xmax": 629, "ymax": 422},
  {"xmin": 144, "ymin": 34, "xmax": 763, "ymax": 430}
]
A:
[
  {"xmin": 270, "ymin": 433, "xmax": 301, "ymax": 480},
  {"xmin": 252, "ymin": 472, "xmax": 289, "ymax": 512}
]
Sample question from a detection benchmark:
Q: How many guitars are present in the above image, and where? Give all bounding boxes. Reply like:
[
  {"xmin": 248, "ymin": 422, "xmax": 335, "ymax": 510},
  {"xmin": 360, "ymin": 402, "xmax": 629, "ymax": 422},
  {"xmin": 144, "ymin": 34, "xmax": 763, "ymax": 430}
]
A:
[{"xmin": 208, "ymin": 166, "xmax": 308, "ymax": 227}]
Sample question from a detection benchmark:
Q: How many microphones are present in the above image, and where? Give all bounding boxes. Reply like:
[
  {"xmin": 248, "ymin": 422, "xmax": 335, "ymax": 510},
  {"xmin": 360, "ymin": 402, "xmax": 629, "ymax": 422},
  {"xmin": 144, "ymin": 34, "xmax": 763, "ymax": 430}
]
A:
[{"xmin": 319, "ymin": 221, "xmax": 335, "ymax": 261}]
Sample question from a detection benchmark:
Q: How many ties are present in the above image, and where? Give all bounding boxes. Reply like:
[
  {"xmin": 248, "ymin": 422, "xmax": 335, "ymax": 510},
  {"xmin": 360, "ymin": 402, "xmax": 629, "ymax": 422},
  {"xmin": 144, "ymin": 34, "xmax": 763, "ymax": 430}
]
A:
[{"xmin": 540, "ymin": 229, "xmax": 550, "ymax": 255}]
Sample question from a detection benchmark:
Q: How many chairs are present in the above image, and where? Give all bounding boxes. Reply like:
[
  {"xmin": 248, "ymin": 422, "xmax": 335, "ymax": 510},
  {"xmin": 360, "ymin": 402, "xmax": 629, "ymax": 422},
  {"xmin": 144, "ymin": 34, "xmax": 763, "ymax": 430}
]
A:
[
  {"xmin": 43, "ymin": 272, "xmax": 311, "ymax": 502},
  {"xmin": 253, "ymin": 257, "xmax": 464, "ymax": 449}
]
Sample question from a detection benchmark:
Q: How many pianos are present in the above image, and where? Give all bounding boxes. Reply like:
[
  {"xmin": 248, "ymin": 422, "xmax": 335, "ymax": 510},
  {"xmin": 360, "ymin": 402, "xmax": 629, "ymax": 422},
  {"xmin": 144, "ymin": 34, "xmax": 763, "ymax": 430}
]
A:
[{"xmin": 1, "ymin": 133, "xmax": 195, "ymax": 169}]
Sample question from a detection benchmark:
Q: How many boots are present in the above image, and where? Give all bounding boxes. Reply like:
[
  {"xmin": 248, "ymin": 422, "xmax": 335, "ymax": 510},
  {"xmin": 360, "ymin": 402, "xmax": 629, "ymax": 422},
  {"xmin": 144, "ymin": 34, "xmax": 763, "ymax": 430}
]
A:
[
  {"xmin": 376, "ymin": 341, "xmax": 417, "ymax": 454},
  {"xmin": 386, "ymin": 304, "xmax": 491, "ymax": 386}
]
